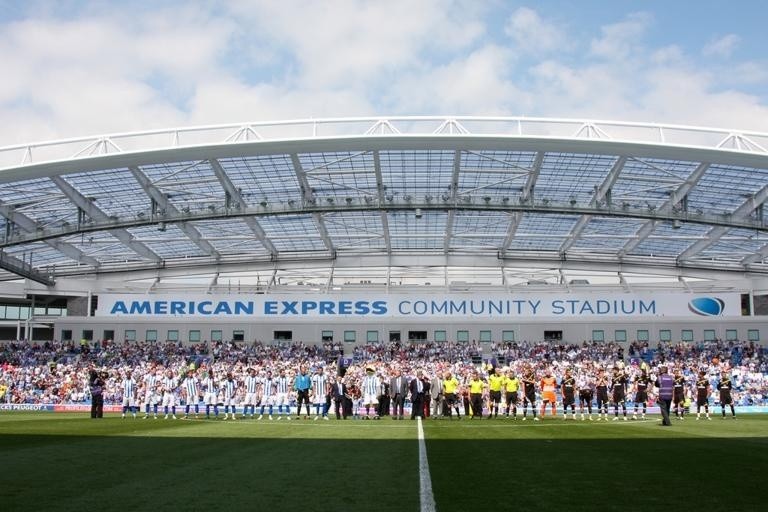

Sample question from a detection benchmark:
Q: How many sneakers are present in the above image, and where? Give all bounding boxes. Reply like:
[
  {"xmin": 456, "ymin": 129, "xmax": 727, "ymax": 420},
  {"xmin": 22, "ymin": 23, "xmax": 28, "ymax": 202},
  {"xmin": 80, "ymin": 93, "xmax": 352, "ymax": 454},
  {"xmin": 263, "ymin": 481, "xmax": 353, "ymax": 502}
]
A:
[{"xmin": 87, "ymin": 413, "xmax": 736, "ymax": 426}]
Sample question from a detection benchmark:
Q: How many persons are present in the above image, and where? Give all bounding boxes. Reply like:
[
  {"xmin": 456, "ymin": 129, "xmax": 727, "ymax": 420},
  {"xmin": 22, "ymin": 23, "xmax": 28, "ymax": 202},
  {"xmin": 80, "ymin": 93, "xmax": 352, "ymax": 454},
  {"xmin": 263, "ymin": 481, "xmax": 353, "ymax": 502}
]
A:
[{"xmin": 0, "ymin": 336, "xmax": 767, "ymax": 426}]
